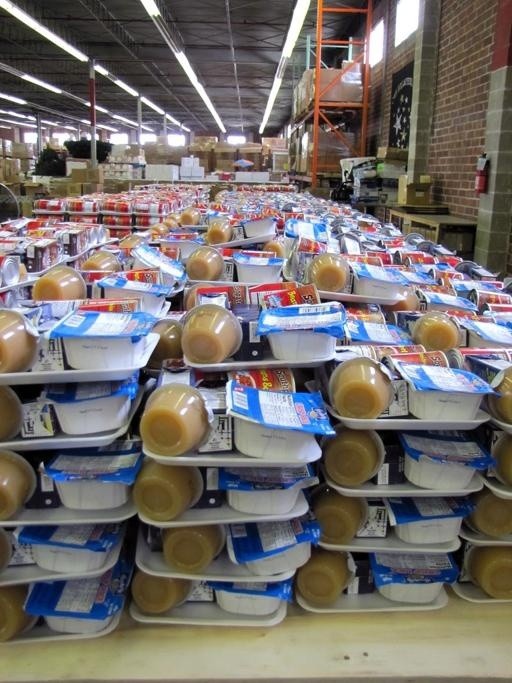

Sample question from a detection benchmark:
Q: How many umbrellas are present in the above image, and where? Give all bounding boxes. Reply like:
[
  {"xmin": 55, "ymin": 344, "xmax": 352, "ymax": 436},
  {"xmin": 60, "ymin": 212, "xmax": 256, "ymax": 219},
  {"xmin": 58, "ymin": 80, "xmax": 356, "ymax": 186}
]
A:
[{"xmin": 231, "ymin": 158, "xmax": 255, "ymax": 168}]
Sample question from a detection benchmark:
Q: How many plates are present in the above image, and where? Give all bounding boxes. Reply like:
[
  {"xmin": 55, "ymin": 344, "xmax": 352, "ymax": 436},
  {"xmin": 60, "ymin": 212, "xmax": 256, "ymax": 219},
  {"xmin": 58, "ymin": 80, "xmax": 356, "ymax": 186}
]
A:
[{"xmin": 2, "ymin": 224, "xmax": 512, "ymax": 647}]
